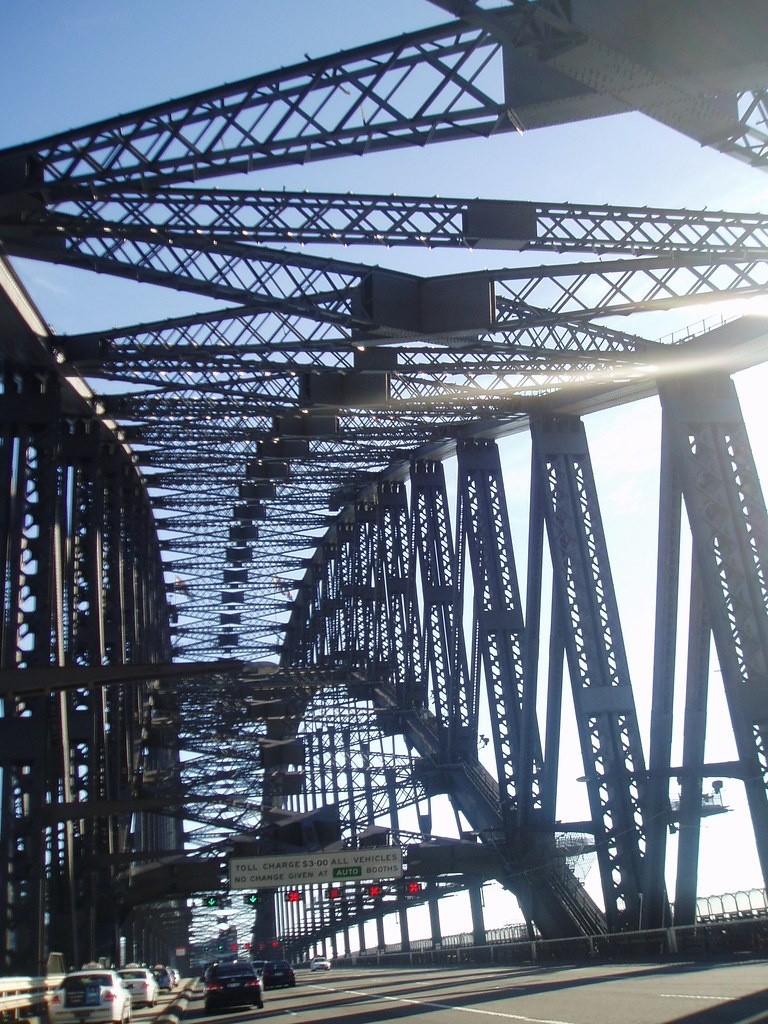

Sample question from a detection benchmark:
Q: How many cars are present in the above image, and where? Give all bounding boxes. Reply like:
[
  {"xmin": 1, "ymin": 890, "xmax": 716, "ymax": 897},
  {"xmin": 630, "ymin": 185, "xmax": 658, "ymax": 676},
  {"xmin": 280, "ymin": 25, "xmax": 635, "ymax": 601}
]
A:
[
  {"xmin": 258, "ymin": 960, "xmax": 295, "ymax": 991},
  {"xmin": 310, "ymin": 956, "xmax": 331, "ymax": 971},
  {"xmin": 115, "ymin": 963, "xmax": 161, "ymax": 1008},
  {"xmin": 47, "ymin": 961, "xmax": 133, "ymax": 1024},
  {"xmin": 147, "ymin": 965, "xmax": 181, "ymax": 991},
  {"xmin": 250, "ymin": 960, "xmax": 269, "ymax": 979},
  {"xmin": 199, "ymin": 959, "xmax": 265, "ymax": 1012}
]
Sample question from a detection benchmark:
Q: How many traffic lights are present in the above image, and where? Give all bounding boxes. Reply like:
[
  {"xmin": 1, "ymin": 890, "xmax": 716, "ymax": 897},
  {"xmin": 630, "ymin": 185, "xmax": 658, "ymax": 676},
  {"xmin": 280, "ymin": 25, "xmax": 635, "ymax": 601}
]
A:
[
  {"xmin": 243, "ymin": 893, "xmax": 263, "ymax": 904},
  {"xmin": 285, "ymin": 891, "xmax": 303, "ymax": 902},
  {"xmin": 325, "ymin": 888, "xmax": 343, "ymax": 898},
  {"xmin": 366, "ymin": 885, "xmax": 384, "ymax": 896},
  {"xmin": 404, "ymin": 881, "xmax": 423, "ymax": 893},
  {"xmin": 206, "ymin": 895, "xmax": 222, "ymax": 907}
]
